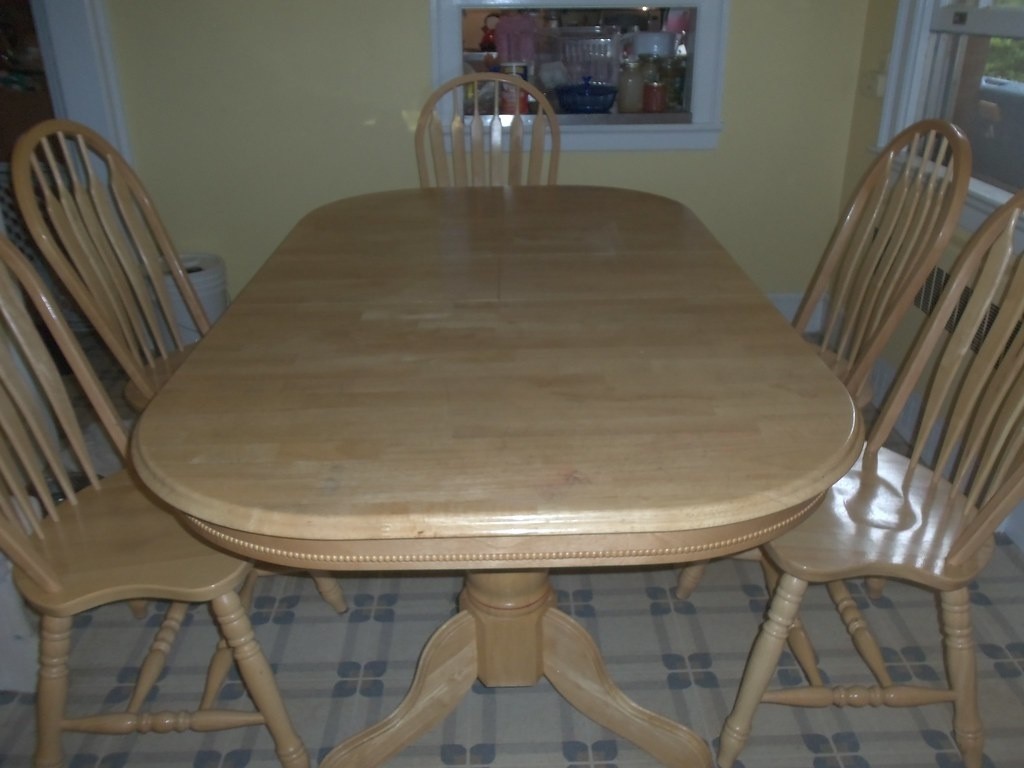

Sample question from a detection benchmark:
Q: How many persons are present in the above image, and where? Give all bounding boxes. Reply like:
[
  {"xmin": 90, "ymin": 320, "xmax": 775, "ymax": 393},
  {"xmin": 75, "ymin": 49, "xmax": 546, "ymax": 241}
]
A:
[{"xmin": 1, "ymin": 1, "xmax": 93, "ymax": 333}]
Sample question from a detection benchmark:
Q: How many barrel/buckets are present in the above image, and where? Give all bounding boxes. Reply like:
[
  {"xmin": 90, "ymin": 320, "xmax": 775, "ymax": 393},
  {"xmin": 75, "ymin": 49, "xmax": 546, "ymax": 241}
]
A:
[{"xmin": 155, "ymin": 254, "xmax": 228, "ymax": 352}]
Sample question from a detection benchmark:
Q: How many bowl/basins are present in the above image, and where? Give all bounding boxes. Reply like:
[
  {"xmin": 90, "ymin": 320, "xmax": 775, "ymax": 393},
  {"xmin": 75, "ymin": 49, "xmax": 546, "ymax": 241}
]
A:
[
  {"xmin": 555, "ymin": 75, "xmax": 617, "ymax": 113},
  {"xmin": 462, "ymin": 52, "xmax": 498, "ymax": 64}
]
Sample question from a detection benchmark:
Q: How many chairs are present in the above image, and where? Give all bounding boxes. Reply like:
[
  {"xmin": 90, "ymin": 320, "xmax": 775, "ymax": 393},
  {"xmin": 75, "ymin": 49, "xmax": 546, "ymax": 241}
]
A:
[
  {"xmin": 0, "ymin": 119, "xmax": 350, "ymax": 767},
  {"xmin": 673, "ymin": 120, "xmax": 1023, "ymax": 768},
  {"xmin": 413, "ymin": 71, "xmax": 562, "ymax": 189}
]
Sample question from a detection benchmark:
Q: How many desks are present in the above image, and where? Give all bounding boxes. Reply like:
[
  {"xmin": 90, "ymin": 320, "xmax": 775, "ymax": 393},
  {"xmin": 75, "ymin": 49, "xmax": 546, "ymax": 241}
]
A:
[{"xmin": 127, "ymin": 185, "xmax": 868, "ymax": 768}]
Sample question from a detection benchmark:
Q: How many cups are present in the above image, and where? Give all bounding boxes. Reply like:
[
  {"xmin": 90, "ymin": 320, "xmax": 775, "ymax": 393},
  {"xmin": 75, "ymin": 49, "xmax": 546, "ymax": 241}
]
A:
[{"xmin": 645, "ymin": 82, "xmax": 665, "ymax": 112}]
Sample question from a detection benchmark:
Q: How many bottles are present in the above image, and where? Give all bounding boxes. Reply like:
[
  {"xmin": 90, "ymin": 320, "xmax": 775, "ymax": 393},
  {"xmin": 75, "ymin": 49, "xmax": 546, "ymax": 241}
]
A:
[
  {"xmin": 640, "ymin": 54, "xmax": 663, "ymax": 83},
  {"xmin": 618, "ymin": 62, "xmax": 644, "ymax": 113}
]
